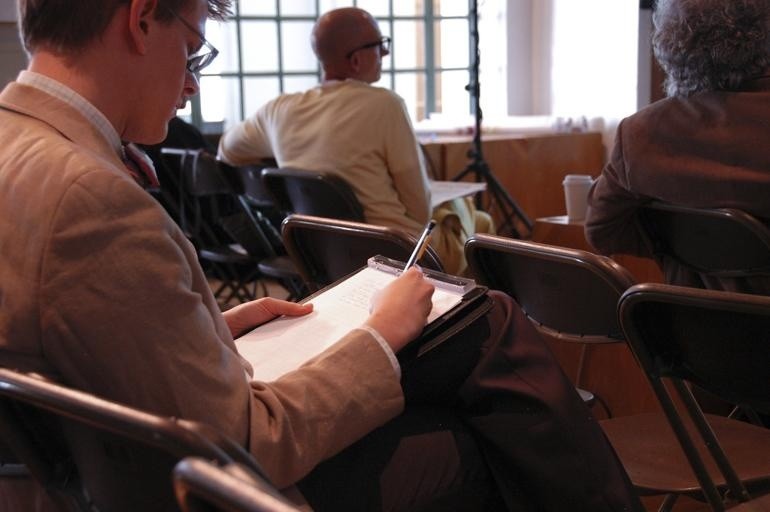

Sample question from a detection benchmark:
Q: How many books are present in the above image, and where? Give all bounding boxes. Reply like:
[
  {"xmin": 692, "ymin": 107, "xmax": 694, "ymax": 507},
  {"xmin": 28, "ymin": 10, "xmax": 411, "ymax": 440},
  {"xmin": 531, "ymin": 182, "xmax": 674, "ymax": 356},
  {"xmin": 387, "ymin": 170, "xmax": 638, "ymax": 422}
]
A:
[
  {"xmin": 428, "ymin": 175, "xmax": 490, "ymax": 209},
  {"xmin": 223, "ymin": 256, "xmax": 494, "ymax": 385}
]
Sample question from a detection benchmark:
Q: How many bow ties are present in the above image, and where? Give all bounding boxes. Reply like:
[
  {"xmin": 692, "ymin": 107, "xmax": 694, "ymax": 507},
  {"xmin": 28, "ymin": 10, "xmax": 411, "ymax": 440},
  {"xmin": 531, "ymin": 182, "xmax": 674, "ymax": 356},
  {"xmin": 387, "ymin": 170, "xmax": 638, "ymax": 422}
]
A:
[{"xmin": 120, "ymin": 142, "xmax": 159, "ymax": 190}]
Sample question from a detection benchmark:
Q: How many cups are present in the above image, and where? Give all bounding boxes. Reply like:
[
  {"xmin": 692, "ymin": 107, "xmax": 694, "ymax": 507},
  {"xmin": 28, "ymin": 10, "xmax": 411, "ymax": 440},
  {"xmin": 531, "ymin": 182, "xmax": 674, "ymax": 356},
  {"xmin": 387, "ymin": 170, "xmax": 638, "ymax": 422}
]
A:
[
  {"xmin": 562, "ymin": 172, "xmax": 595, "ymax": 223},
  {"xmin": 591, "ymin": 117, "xmax": 605, "ymax": 132}
]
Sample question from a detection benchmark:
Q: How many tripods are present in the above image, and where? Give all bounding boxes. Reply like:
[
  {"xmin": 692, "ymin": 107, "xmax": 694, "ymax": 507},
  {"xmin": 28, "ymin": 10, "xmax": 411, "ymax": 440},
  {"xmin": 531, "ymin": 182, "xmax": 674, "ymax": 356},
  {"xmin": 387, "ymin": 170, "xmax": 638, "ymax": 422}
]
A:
[{"xmin": 448, "ymin": 1, "xmax": 534, "ymax": 240}]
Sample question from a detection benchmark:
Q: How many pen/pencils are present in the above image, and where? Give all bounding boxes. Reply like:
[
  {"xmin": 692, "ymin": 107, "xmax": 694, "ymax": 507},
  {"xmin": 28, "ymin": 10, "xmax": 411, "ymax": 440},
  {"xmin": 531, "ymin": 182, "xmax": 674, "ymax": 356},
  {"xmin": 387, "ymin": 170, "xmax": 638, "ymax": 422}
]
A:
[{"xmin": 402, "ymin": 219, "xmax": 437, "ymax": 275}]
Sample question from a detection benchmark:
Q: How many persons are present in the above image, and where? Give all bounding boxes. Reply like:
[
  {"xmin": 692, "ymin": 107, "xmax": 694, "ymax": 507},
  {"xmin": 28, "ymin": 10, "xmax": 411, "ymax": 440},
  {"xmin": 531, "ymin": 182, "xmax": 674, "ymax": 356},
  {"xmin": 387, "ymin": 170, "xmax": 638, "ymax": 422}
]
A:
[
  {"xmin": 0, "ymin": 1, "xmax": 645, "ymax": 512},
  {"xmin": 585, "ymin": 0, "xmax": 770, "ymax": 292},
  {"xmin": 219, "ymin": 7, "xmax": 497, "ymax": 281}
]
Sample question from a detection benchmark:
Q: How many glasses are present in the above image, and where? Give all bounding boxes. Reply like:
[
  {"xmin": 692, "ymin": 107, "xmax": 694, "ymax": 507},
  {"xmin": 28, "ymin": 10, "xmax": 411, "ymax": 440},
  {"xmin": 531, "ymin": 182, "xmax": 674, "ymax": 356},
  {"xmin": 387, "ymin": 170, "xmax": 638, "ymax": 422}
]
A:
[
  {"xmin": 346, "ymin": 36, "xmax": 392, "ymax": 63},
  {"xmin": 158, "ymin": 0, "xmax": 219, "ymax": 71}
]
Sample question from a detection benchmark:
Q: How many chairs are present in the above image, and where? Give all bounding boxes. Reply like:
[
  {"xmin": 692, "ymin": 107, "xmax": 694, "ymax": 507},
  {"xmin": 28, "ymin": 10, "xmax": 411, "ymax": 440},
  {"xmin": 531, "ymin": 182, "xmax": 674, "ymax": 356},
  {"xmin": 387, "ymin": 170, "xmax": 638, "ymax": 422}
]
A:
[
  {"xmin": 461, "ymin": 202, "xmax": 769, "ymax": 512},
  {"xmin": 1, "ymin": 366, "xmax": 304, "ymax": 512},
  {"xmin": 159, "ymin": 147, "xmax": 368, "ymax": 308}
]
